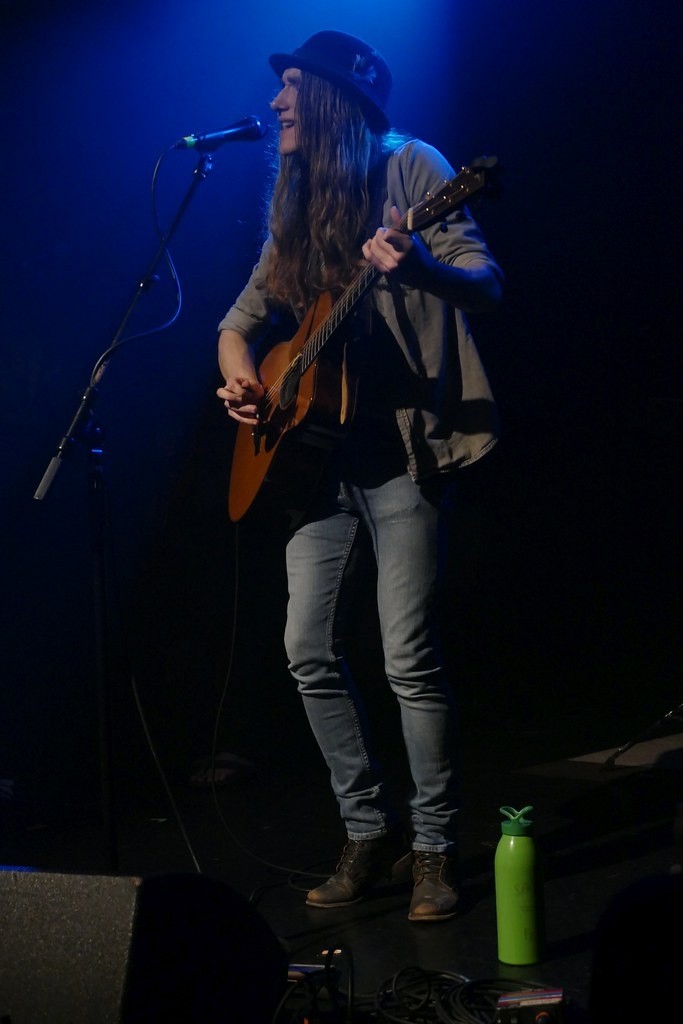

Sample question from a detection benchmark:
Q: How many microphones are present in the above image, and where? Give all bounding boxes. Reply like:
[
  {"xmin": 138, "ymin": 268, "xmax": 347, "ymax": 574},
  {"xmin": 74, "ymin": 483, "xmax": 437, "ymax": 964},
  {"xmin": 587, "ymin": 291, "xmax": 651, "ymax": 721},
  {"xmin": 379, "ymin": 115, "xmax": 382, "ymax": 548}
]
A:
[{"xmin": 174, "ymin": 114, "xmax": 269, "ymax": 152}]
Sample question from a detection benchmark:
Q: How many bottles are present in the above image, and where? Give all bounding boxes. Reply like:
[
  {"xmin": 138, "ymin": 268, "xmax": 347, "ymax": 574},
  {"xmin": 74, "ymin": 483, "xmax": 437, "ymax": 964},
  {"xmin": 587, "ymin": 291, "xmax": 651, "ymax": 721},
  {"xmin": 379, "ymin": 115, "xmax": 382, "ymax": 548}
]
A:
[{"xmin": 494, "ymin": 805, "xmax": 538, "ymax": 966}]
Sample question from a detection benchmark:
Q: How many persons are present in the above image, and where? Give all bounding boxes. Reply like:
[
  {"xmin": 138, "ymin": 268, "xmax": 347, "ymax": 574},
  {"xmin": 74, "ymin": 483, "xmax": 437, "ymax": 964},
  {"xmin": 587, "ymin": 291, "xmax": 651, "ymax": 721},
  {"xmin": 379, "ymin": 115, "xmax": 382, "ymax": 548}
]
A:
[{"xmin": 210, "ymin": 36, "xmax": 511, "ymax": 922}]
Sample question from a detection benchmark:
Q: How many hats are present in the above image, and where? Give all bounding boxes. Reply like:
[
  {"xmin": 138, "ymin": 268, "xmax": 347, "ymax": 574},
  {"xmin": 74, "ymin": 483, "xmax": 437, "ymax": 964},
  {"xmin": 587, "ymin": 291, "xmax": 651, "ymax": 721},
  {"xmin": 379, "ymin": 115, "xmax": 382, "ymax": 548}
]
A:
[{"xmin": 269, "ymin": 29, "xmax": 394, "ymax": 135}]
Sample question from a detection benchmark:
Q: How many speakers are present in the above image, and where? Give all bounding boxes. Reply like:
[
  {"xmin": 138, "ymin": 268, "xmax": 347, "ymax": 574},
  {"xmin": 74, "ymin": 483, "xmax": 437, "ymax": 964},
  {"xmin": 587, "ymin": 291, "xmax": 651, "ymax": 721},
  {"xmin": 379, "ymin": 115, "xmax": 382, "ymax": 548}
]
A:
[{"xmin": 2, "ymin": 867, "xmax": 151, "ymax": 1024}]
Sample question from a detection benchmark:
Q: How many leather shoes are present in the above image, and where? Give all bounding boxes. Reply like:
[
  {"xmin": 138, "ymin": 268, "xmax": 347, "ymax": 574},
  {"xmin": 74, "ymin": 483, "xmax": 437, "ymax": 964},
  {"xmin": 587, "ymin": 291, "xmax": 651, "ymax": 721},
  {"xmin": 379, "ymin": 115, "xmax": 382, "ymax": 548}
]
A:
[
  {"xmin": 304, "ymin": 820, "xmax": 412, "ymax": 908},
  {"xmin": 408, "ymin": 842, "xmax": 466, "ymax": 922}
]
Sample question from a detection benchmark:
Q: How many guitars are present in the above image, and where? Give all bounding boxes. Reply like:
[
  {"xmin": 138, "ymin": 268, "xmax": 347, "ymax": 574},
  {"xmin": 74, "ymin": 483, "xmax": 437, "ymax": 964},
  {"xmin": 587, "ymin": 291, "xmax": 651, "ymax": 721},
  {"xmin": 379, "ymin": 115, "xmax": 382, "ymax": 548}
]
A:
[{"xmin": 228, "ymin": 154, "xmax": 498, "ymax": 522}]
